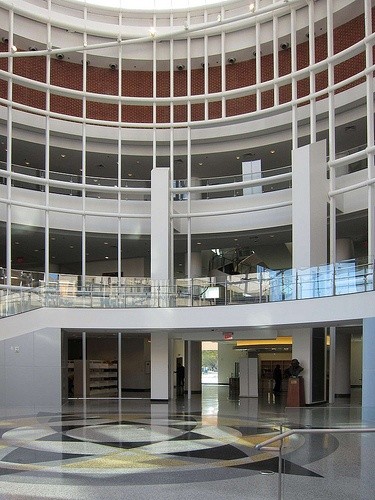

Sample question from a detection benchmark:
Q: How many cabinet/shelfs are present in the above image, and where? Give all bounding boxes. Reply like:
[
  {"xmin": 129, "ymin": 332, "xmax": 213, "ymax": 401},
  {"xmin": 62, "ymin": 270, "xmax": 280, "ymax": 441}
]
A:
[{"xmin": 74, "ymin": 358, "xmax": 118, "ymax": 398}]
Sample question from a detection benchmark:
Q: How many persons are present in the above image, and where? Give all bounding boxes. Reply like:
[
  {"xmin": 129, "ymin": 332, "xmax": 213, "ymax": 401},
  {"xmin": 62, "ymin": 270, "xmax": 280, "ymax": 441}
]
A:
[
  {"xmin": 273, "ymin": 364, "xmax": 282, "ymax": 398},
  {"xmin": 178, "ymin": 363, "xmax": 185, "ymax": 389},
  {"xmin": 287, "ymin": 358, "xmax": 304, "ymax": 377}
]
